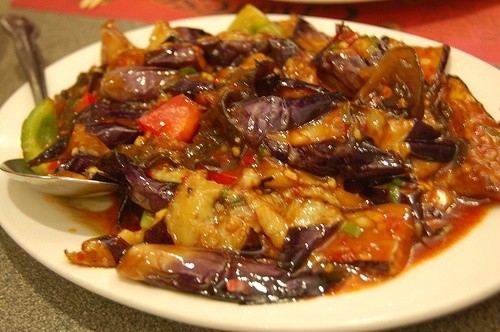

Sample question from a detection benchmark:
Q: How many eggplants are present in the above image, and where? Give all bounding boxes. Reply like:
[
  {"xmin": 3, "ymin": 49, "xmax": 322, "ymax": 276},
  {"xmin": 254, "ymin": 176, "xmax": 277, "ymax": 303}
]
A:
[{"xmin": 22, "ymin": 3, "xmax": 500, "ymax": 305}]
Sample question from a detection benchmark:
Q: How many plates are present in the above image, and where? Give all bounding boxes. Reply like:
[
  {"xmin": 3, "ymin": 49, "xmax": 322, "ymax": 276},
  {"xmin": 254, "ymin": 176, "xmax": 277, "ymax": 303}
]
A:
[{"xmin": 0, "ymin": 13, "xmax": 499, "ymax": 332}]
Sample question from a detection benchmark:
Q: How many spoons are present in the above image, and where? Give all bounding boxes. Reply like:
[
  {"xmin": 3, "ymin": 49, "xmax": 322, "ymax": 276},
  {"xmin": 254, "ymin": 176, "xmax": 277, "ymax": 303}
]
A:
[{"xmin": 0, "ymin": 13, "xmax": 119, "ymax": 197}]
